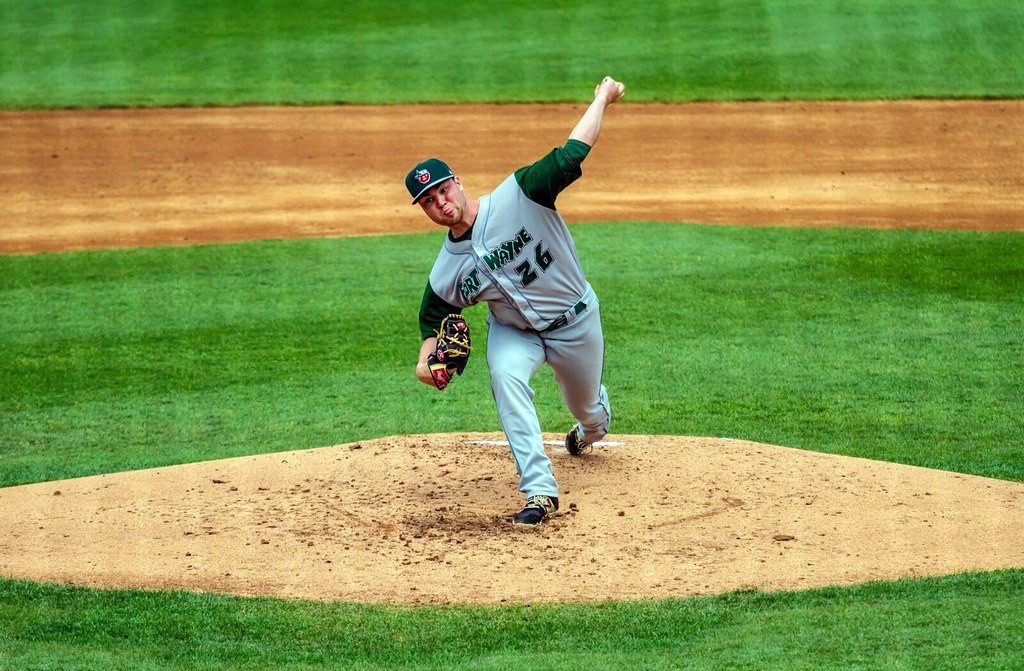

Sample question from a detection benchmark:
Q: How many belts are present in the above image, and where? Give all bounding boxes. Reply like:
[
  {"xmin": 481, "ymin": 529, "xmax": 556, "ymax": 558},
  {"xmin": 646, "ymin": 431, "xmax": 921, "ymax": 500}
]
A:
[{"xmin": 533, "ymin": 301, "xmax": 586, "ymax": 332}]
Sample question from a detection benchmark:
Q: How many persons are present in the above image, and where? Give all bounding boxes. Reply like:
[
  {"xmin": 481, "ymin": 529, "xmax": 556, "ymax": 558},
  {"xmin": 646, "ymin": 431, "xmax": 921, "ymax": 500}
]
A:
[{"xmin": 405, "ymin": 75, "xmax": 627, "ymax": 530}]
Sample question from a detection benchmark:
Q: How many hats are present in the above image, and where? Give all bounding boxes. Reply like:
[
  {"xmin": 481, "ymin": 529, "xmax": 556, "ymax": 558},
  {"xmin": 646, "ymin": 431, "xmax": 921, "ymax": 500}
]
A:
[{"xmin": 405, "ymin": 158, "xmax": 455, "ymax": 205}]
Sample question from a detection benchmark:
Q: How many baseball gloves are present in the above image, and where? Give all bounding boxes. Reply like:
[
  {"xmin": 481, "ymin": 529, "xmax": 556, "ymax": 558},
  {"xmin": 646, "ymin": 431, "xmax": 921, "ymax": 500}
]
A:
[{"xmin": 427, "ymin": 313, "xmax": 474, "ymax": 391}]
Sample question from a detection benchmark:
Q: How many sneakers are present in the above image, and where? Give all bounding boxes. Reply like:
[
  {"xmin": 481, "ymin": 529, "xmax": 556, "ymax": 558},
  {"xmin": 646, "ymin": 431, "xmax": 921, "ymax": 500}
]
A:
[
  {"xmin": 565, "ymin": 424, "xmax": 594, "ymax": 456},
  {"xmin": 512, "ymin": 495, "xmax": 559, "ymax": 528}
]
